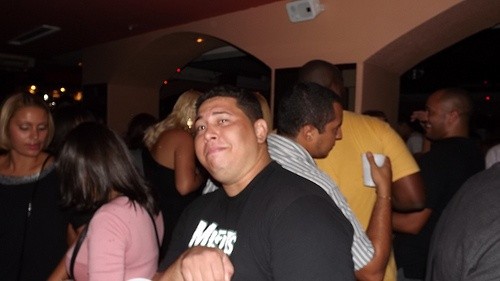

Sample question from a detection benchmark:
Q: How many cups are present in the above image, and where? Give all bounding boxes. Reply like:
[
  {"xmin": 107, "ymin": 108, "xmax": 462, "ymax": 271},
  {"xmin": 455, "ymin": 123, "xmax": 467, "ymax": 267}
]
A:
[{"xmin": 362, "ymin": 153, "xmax": 385, "ymax": 188}]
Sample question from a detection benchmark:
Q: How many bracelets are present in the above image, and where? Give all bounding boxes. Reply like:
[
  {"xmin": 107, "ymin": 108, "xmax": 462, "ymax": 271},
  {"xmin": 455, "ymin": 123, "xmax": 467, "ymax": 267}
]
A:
[{"xmin": 377, "ymin": 192, "xmax": 392, "ymax": 201}]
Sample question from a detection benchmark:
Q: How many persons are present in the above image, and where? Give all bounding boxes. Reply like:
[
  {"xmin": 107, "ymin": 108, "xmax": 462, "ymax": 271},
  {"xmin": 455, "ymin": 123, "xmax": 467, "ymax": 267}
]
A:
[
  {"xmin": 0, "ymin": 91, "xmax": 89, "ymax": 281},
  {"xmin": 299, "ymin": 60, "xmax": 486, "ymax": 281},
  {"xmin": 46, "ymin": 120, "xmax": 165, "ymax": 281},
  {"xmin": 135, "ymin": 89, "xmax": 204, "ymax": 269},
  {"xmin": 203, "ymin": 81, "xmax": 392, "ymax": 281},
  {"xmin": 151, "ymin": 84, "xmax": 356, "ymax": 281},
  {"xmin": 424, "ymin": 162, "xmax": 500, "ymax": 281}
]
